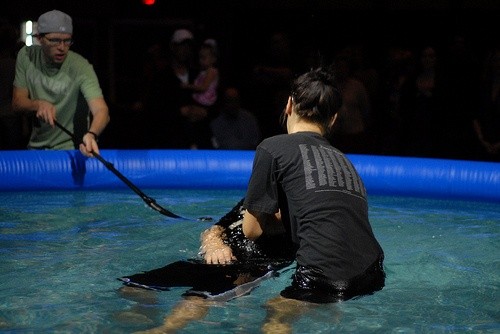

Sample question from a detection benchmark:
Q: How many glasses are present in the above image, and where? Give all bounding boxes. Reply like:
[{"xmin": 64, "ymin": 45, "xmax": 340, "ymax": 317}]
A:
[{"xmin": 44, "ymin": 37, "xmax": 73, "ymax": 46}]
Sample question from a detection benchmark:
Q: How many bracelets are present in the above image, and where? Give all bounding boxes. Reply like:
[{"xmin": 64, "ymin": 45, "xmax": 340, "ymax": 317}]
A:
[{"xmin": 86, "ymin": 130, "xmax": 99, "ymax": 143}]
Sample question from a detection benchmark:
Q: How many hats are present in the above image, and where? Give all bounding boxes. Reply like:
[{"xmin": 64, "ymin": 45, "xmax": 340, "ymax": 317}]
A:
[
  {"xmin": 173, "ymin": 29, "xmax": 193, "ymax": 43},
  {"xmin": 32, "ymin": 10, "xmax": 73, "ymax": 37}
]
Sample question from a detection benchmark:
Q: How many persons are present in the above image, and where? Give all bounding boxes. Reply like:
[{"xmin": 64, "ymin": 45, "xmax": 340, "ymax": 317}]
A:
[
  {"xmin": 242, "ymin": 69, "xmax": 386, "ymax": 334},
  {"xmin": 93, "ymin": 24, "xmax": 500, "ymax": 166},
  {"xmin": 113, "ymin": 181, "xmax": 297, "ymax": 334},
  {"xmin": 12, "ymin": 9, "xmax": 112, "ymax": 158}
]
